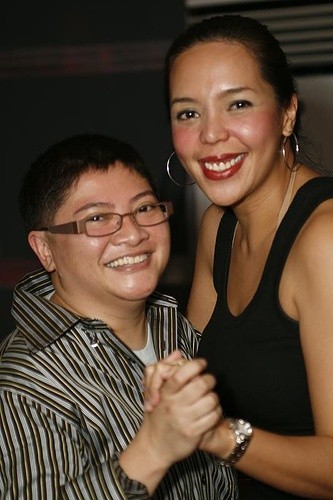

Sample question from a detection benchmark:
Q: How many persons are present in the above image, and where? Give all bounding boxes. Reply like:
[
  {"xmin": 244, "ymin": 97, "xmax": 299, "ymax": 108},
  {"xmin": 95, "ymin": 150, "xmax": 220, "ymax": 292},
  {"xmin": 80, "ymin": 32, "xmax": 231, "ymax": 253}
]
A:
[
  {"xmin": 136, "ymin": 11, "xmax": 333, "ymax": 500},
  {"xmin": 0, "ymin": 126, "xmax": 240, "ymax": 499}
]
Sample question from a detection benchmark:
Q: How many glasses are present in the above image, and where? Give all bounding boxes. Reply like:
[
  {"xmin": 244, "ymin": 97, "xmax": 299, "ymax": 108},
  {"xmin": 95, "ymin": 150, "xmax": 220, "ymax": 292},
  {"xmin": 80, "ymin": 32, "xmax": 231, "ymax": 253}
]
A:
[{"xmin": 42, "ymin": 200, "xmax": 175, "ymax": 238}]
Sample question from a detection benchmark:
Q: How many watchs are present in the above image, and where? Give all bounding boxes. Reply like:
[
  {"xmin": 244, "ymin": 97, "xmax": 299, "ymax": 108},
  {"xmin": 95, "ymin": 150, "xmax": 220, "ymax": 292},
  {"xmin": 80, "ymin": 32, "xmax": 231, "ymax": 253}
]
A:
[{"xmin": 215, "ymin": 416, "xmax": 254, "ymax": 471}]
